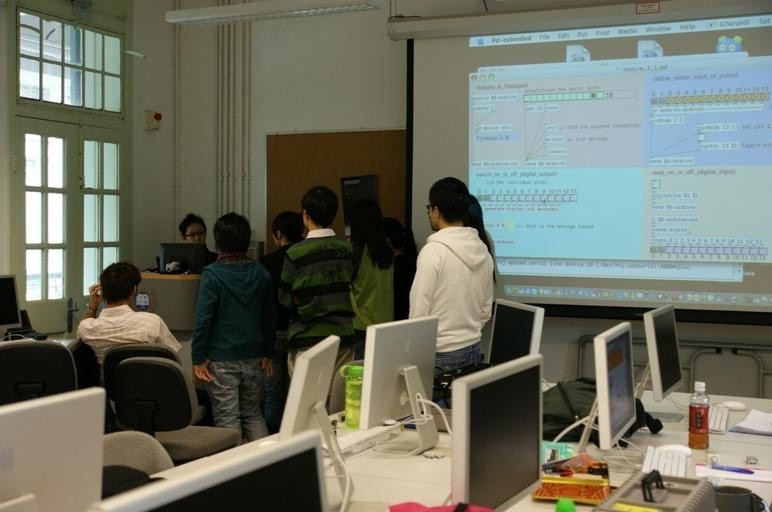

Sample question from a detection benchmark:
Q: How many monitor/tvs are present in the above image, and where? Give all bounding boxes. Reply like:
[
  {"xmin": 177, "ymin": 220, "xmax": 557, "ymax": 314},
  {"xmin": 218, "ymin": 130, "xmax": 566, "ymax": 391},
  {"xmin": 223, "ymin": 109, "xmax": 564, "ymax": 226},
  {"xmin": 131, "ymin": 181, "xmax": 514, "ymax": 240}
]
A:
[
  {"xmin": 632, "ymin": 304, "xmax": 686, "ymax": 423},
  {"xmin": 576, "ymin": 320, "xmax": 644, "ymax": 472},
  {"xmin": 452, "ymin": 353, "xmax": 545, "ymax": 512},
  {"xmin": 487, "ymin": 299, "xmax": 545, "ymax": 367},
  {"xmin": 89, "ymin": 430, "xmax": 332, "ymax": 512},
  {"xmin": 160, "ymin": 242, "xmax": 207, "ymax": 276},
  {"xmin": 0, "ymin": 274, "xmax": 23, "ymax": 344},
  {"xmin": 275, "ymin": 335, "xmax": 354, "ymax": 512},
  {"xmin": 358, "ymin": 315, "xmax": 440, "ymax": 456}
]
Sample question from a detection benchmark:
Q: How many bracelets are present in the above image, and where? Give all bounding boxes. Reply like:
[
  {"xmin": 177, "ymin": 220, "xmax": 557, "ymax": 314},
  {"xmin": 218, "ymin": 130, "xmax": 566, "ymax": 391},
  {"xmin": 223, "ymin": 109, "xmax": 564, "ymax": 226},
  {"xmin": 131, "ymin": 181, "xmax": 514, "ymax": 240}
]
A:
[{"xmin": 85, "ymin": 303, "xmax": 94, "ymax": 314}]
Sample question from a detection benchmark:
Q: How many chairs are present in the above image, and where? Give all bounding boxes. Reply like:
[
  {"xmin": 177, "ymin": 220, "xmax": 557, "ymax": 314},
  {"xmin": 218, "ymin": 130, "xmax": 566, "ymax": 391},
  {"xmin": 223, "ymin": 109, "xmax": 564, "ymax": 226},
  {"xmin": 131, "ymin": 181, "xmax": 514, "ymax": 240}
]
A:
[
  {"xmin": 66, "ymin": 341, "xmax": 113, "ymax": 424},
  {"xmin": 114, "ymin": 356, "xmax": 240, "ymax": 460},
  {"xmin": 102, "ymin": 431, "xmax": 177, "ymax": 476},
  {"xmin": 1, "ymin": 341, "xmax": 78, "ymax": 405},
  {"xmin": 101, "ymin": 343, "xmax": 205, "ymax": 426}
]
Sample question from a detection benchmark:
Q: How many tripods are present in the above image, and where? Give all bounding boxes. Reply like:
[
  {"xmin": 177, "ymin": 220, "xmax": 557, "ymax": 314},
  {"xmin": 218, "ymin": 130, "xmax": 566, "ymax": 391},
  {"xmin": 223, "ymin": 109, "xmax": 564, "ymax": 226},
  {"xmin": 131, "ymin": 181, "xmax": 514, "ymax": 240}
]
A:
[{"xmin": 0, "ymin": 387, "xmax": 111, "ymax": 511}]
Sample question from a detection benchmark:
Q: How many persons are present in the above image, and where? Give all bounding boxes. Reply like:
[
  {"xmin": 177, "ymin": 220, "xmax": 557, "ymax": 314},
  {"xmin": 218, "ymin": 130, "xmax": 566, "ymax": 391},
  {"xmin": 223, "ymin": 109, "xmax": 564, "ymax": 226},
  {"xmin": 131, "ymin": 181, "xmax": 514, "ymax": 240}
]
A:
[
  {"xmin": 260, "ymin": 210, "xmax": 305, "ymax": 435},
  {"xmin": 344, "ymin": 199, "xmax": 394, "ymax": 361},
  {"xmin": 75, "ymin": 262, "xmax": 182, "ymax": 427},
  {"xmin": 193, "ymin": 212, "xmax": 273, "ymax": 444},
  {"xmin": 409, "ymin": 176, "xmax": 495, "ymax": 410},
  {"xmin": 466, "ymin": 192, "xmax": 502, "ymax": 363},
  {"xmin": 277, "ymin": 184, "xmax": 354, "ymax": 401},
  {"xmin": 382, "ymin": 218, "xmax": 416, "ymax": 325},
  {"xmin": 179, "ymin": 214, "xmax": 218, "ymax": 270}
]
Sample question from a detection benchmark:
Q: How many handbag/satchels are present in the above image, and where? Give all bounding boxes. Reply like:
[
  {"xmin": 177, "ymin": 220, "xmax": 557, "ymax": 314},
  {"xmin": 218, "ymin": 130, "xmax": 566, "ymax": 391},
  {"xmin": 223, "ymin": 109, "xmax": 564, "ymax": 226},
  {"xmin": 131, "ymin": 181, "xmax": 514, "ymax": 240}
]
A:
[{"xmin": 543, "ymin": 378, "xmax": 662, "ymax": 447}]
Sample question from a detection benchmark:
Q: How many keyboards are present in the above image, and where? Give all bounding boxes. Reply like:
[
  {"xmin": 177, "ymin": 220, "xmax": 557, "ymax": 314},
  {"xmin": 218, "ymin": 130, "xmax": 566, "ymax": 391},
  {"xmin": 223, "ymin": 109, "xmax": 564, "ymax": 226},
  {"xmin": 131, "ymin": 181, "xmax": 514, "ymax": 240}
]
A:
[
  {"xmin": 640, "ymin": 445, "xmax": 695, "ymax": 481},
  {"xmin": 323, "ymin": 426, "xmax": 393, "ymax": 458},
  {"xmin": 706, "ymin": 406, "xmax": 729, "ymax": 434}
]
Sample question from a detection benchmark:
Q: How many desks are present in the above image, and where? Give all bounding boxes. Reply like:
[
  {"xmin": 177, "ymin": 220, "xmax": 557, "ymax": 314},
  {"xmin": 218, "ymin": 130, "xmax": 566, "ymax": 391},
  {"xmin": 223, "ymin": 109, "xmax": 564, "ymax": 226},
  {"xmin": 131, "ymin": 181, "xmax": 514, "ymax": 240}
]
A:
[{"xmin": 146, "ymin": 379, "xmax": 772, "ymax": 512}]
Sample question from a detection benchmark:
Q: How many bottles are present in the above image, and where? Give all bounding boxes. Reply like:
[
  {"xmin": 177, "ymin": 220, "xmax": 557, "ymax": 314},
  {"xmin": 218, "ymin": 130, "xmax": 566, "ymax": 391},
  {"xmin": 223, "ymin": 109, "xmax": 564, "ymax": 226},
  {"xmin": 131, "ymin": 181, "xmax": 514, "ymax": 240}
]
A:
[
  {"xmin": 688, "ymin": 381, "xmax": 709, "ymax": 450},
  {"xmin": 344, "ymin": 364, "xmax": 363, "ymax": 429}
]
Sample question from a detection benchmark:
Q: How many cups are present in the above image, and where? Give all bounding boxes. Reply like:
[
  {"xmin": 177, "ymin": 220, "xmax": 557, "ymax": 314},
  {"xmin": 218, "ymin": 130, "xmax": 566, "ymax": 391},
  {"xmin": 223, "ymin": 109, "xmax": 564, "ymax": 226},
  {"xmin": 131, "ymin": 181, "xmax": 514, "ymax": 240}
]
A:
[{"xmin": 712, "ymin": 484, "xmax": 751, "ymax": 511}]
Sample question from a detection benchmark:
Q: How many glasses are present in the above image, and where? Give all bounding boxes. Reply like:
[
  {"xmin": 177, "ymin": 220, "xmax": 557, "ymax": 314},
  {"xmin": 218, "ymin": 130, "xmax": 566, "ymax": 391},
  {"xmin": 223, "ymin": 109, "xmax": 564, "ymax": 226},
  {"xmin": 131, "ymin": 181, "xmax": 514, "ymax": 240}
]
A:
[{"xmin": 184, "ymin": 230, "xmax": 206, "ymax": 235}]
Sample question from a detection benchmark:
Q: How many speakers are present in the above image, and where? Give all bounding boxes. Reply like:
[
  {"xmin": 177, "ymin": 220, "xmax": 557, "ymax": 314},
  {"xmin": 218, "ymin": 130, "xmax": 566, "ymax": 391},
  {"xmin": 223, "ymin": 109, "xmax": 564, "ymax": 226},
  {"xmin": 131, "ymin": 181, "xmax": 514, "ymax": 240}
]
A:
[{"xmin": 340, "ymin": 174, "xmax": 383, "ymax": 238}]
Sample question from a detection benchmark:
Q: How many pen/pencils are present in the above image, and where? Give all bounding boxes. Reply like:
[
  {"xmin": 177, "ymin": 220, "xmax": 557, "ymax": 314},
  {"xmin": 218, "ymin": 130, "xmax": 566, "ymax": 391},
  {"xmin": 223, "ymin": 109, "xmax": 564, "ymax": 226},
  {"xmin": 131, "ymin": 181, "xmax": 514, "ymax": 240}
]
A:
[{"xmin": 710, "ymin": 463, "xmax": 754, "ymax": 473}]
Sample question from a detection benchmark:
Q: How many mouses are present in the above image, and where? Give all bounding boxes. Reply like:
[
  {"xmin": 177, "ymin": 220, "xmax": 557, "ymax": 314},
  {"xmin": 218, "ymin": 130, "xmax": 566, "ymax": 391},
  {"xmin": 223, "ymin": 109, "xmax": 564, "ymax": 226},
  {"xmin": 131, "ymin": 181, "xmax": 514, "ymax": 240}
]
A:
[
  {"xmin": 723, "ymin": 400, "xmax": 746, "ymax": 410},
  {"xmin": 663, "ymin": 443, "xmax": 692, "ymax": 456}
]
